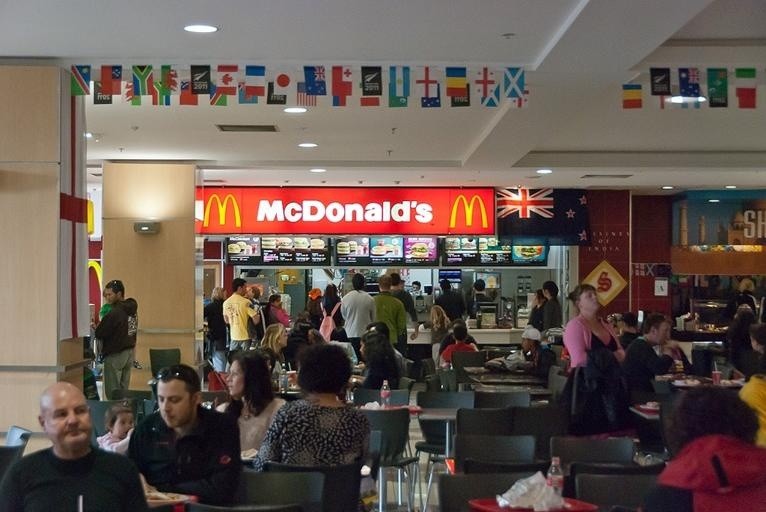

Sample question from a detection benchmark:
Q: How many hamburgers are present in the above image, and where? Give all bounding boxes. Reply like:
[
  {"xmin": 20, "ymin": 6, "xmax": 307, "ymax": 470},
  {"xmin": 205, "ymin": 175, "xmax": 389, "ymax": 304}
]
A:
[
  {"xmin": 370, "ymin": 244, "xmax": 393, "ymax": 255},
  {"xmin": 411, "ymin": 243, "xmax": 430, "ymax": 256},
  {"xmin": 226, "ymin": 241, "xmax": 246, "ymax": 253},
  {"xmin": 336, "ymin": 241, "xmax": 356, "ymax": 255},
  {"xmin": 445, "ymin": 238, "xmax": 499, "ymax": 249},
  {"xmin": 262, "ymin": 237, "xmax": 326, "ymax": 250},
  {"xmin": 520, "ymin": 246, "xmax": 538, "ymax": 257}
]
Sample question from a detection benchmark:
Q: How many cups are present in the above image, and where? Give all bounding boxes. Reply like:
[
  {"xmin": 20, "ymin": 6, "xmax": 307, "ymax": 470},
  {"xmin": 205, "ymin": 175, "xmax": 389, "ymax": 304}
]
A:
[
  {"xmin": 286, "ymin": 370, "xmax": 296, "ymax": 385},
  {"xmin": 250, "ymin": 243, "xmax": 258, "ymax": 253},
  {"xmin": 711, "ymin": 370, "xmax": 722, "ymax": 386}
]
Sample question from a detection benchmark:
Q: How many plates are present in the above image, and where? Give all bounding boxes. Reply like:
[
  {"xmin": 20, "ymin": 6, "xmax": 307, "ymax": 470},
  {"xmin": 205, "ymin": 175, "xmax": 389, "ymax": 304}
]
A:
[
  {"xmin": 144, "ymin": 493, "xmax": 188, "ymax": 506},
  {"xmin": 468, "ymin": 493, "xmax": 600, "ymax": 511}
]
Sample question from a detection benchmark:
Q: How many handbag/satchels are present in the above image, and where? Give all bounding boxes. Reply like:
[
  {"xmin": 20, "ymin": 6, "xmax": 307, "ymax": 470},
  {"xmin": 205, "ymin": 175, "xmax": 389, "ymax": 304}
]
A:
[
  {"xmin": 581, "ymin": 378, "xmax": 629, "ymax": 428},
  {"xmin": 484, "ymin": 351, "xmax": 525, "ymax": 372},
  {"xmin": 631, "ymin": 451, "xmax": 664, "ymax": 476}
]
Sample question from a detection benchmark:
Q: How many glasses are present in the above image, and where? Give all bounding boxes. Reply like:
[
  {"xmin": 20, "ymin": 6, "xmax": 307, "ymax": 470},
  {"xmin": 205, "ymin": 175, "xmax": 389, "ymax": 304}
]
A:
[{"xmin": 156, "ymin": 367, "xmax": 184, "ymax": 379}]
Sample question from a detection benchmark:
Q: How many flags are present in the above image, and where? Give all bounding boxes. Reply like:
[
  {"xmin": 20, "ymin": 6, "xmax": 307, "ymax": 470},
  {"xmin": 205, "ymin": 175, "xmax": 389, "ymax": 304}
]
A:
[
  {"xmin": 66, "ymin": 59, "xmax": 530, "ymax": 113},
  {"xmin": 621, "ymin": 65, "xmax": 757, "ymax": 111},
  {"xmin": 494, "ymin": 186, "xmax": 594, "ymax": 247}
]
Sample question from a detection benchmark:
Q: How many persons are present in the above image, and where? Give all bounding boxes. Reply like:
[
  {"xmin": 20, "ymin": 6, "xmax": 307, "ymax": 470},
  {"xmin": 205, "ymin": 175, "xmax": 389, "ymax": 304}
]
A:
[{"xmin": 0, "ymin": 269, "xmax": 766, "ymax": 512}]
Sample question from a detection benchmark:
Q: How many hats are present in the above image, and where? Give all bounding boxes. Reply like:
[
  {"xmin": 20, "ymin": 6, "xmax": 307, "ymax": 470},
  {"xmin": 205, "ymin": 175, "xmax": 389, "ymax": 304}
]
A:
[{"xmin": 521, "ymin": 325, "xmax": 541, "ymax": 342}]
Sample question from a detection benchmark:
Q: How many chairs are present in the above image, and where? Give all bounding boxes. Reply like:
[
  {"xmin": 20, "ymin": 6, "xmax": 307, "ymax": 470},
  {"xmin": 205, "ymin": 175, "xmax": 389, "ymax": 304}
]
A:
[
  {"xmin": 0, "ymin": 425, "xmax": 696, "ymax": 512},
  {"xmin": 84, "ymin": 345, "xmax": 735, "ymax": 429}
]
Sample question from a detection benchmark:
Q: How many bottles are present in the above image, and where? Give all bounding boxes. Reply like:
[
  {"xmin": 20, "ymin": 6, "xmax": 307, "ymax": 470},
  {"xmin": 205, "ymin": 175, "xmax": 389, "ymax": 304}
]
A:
[
  {"xmin": 279, "ymin": 368, "xmax": 288, "ymax": 393},
  {"xmin": 379, "ymin": 380, "xmax": 390, "ymax": 406},
  {"xmin": 548, "ymin": 456, "xmax": 562, "ymax": 502},
  {"xmin": 475, "ymin": 310, "xmax": 482, "ymax": 329}
]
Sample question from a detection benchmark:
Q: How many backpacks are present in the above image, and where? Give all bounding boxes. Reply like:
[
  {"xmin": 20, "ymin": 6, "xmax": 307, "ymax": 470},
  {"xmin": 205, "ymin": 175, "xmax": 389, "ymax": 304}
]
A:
[{"xmin": 318, "ymin": 302, "xmax": 341, "ymax": 342}]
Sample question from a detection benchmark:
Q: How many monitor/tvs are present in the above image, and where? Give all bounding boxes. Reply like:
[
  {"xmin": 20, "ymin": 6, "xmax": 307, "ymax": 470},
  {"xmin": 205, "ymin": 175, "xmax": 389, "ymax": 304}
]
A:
[{"xmin": 439, "ymin": 269, "xmax": 461, "ymax": 283}]
